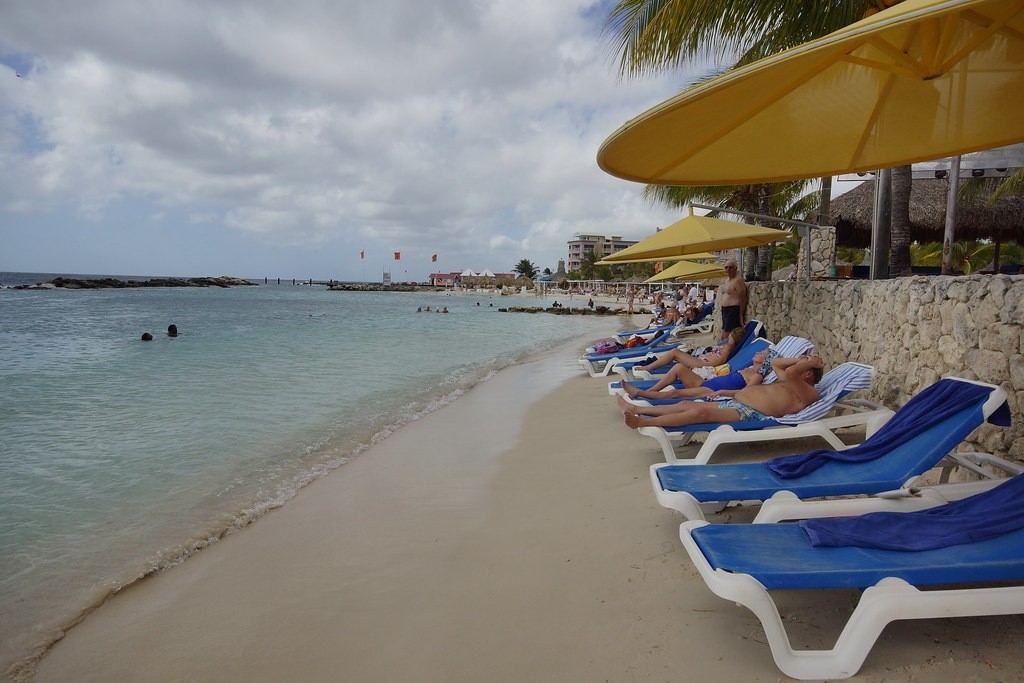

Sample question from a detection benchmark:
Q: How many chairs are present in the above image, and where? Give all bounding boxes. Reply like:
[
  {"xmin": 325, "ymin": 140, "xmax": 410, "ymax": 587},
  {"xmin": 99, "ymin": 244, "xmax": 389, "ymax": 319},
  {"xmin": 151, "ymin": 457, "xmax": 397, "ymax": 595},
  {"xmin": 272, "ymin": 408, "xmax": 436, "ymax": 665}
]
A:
[{"xmin": 578, "ymin": 304, "xmax": 1024, "ymax": 680}]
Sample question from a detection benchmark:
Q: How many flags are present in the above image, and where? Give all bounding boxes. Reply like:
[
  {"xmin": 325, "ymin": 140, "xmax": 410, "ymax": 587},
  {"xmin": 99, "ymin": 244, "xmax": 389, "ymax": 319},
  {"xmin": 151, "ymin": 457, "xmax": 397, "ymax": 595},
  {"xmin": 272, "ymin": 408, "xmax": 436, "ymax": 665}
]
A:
[
  {"xmin": 360, "ymin": 251, "xmax": 365, "ymax": 258},
  {"xmin": 395, "ymin": 252, "xmax": 399, "ymax": 261},
  {"xmin": 432, "ymin": 254, "xmax": 437, "ymax": 262}
]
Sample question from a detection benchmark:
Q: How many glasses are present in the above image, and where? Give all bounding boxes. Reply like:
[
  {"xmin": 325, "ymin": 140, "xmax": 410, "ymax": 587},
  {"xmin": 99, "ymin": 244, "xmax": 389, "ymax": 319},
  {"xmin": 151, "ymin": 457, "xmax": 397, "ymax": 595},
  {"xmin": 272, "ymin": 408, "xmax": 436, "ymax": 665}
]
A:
[{"xmin": 725, "ymin": 265, "xmax": 737, "ymax": 269}]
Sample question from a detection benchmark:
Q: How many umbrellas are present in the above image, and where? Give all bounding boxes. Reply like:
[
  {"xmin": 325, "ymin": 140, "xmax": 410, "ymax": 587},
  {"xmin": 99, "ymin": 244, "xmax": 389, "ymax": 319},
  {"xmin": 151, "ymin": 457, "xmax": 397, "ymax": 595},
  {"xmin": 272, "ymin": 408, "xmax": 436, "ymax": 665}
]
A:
[{"xmin": 595, "ymin": 0, "xmax": 1024, "ymax": 284}]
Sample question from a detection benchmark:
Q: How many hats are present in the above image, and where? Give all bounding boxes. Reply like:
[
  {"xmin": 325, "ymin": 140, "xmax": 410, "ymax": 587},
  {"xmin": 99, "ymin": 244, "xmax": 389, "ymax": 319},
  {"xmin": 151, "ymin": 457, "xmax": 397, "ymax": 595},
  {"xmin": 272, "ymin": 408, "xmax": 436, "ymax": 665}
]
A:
[{"xmin": 758, "ymin": 349, "xmax": 782, "ymax": 377}]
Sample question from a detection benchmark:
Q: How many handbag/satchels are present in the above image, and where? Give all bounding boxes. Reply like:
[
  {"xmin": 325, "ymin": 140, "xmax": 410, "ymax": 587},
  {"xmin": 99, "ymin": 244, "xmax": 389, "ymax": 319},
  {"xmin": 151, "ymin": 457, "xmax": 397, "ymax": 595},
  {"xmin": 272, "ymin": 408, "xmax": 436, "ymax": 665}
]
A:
[{"xmin": 596, "ymin": 341, "xmax": 618, "ymax": 354}]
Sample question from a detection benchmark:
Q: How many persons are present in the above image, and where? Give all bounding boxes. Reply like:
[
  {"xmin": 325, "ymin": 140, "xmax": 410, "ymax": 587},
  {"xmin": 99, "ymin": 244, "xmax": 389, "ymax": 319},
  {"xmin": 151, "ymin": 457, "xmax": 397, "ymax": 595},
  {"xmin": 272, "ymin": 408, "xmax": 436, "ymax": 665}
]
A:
[
  {"xmin": 417, "ymin": 260, "xmax": 823, "ymax": 429},
  {"xmin": 168, "ymin": 325, "xmax": 177, "ymax": 336},
  {"xmin": 141, "ymin": 333, "xmax": 152, "ymax": 340}
]
